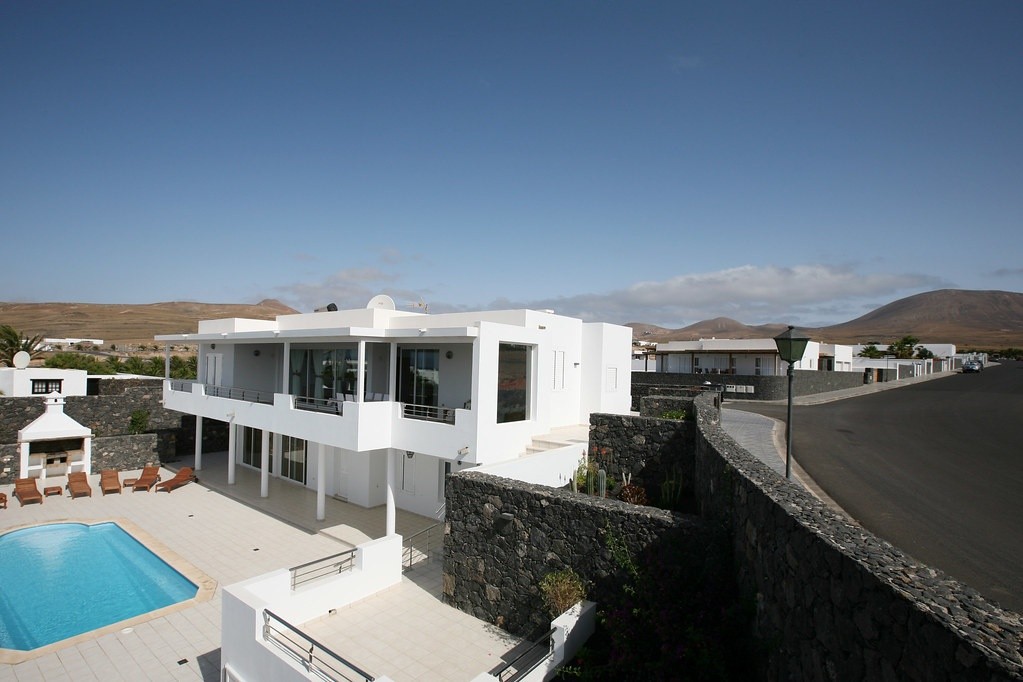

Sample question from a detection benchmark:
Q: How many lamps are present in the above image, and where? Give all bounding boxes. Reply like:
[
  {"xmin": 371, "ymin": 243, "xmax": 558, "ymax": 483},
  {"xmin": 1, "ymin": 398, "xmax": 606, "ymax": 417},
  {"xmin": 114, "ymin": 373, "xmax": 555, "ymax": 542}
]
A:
[{"xmin": 406, "ymin": 451, "xmax": 414, "ymax": 458}]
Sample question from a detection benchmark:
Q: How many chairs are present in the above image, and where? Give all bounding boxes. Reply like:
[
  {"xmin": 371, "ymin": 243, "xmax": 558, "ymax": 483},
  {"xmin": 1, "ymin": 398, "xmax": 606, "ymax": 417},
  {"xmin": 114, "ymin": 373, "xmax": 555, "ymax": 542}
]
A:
[
  {"xmin": 0, "ymin": 493, "xmax": 8, "ymax": 509},
  {"xmin": 373, "ymin": 392, "xmax": 383, "ymax": 401},
  {"xmin": 365, "ymin": 392, "xmax": 374, "ymax": 400},
  {"xmin": 345, "ymin": 394, "xmax": 353, "ymax": 402},
  {"xmin": 354, "ymin": 395, "xmax": 357, "ymax": 401},
  {"xmin": 11, "ymin": 477, "xmax": 43, "ymax": 507},
  {"xmin": 382, "ymin": 393, "xmax": 389, "ymax": 401},
  {"xmin": 336, "ymin": 392, "xmax": 344, "ymax": 413},
  {"xmin": 132, "ymin": 466, "xmax": 161, "ymax": 493},
  {"xmin": 155, "ymin": 466, "xmax": 199, "ymax": 493},
  {"xmin": 99, "ymin": 468, "xmax": 122, "ymax": 497},
  {"xmin": 65, "ymin": 472, "xmax": 91, "ymax": 500}
]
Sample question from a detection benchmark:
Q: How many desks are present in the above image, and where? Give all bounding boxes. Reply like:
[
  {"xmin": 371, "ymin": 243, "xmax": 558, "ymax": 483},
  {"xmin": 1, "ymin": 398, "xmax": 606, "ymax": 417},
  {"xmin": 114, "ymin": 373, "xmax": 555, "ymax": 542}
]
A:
[
  {"xmin": 44, "ymin": 486, "xmax": 62, "ymax": 497},
  {"xmin": 123, "ymin": 478, "xmax": 138, "ymax": 488}
]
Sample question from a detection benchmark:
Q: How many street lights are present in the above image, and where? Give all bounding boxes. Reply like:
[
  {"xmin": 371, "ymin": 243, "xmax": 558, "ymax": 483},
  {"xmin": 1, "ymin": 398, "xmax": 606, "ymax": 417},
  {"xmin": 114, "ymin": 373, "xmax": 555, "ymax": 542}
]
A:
[{"xmin": 772, "ymin": 325, "xmax": 811, "ymax": 478}]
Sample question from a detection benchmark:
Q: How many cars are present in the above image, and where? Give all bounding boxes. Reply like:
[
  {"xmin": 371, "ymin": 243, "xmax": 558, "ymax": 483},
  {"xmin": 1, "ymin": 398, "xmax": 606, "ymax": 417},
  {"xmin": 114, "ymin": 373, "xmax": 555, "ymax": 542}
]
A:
[{"xmin": 962, "ymin": 360, "xmax": 983, "ymax": 372}]
